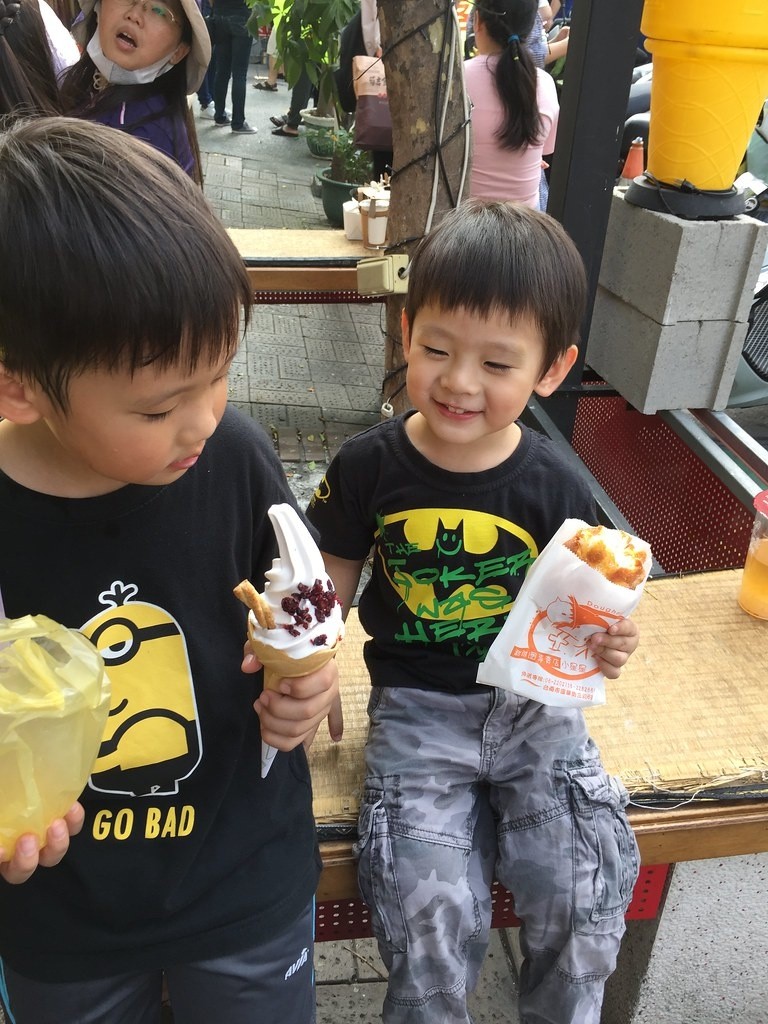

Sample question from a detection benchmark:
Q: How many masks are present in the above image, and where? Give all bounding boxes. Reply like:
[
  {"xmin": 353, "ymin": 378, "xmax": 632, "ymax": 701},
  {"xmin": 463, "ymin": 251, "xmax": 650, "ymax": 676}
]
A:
[{"xmin": 86, "ymin": 3, "xmax": 182, "ymax": 85}]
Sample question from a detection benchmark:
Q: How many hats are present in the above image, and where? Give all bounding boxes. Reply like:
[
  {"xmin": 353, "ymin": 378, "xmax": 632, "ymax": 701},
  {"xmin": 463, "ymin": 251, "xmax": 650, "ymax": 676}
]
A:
[{"xmin": 73, "ymin": 1, "xmax": 211, "ymax": 96}]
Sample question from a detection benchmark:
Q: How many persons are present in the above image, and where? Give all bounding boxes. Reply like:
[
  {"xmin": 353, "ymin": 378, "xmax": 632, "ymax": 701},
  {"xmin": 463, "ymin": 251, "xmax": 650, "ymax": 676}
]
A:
[
  {"xmin": 302, "ymin": 201, "xmax": 641, "ymax": 1024},
  {"xmin": 200, "ymin": 0, "xmax": 570, "ymax": 217},
  {"xmin": 3, "ymin": 114, "xmax": 340, "ymax": 1022},
  {"xmin": 56, "ymin": 0, "xmax": 214, "ymax": 191}
]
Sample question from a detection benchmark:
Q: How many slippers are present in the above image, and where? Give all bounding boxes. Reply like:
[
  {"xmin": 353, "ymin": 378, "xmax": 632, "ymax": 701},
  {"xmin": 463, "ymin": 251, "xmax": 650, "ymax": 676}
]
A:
[{"xmin": 272, "ymin": 124, "xmax": 300, "ymax": 136}]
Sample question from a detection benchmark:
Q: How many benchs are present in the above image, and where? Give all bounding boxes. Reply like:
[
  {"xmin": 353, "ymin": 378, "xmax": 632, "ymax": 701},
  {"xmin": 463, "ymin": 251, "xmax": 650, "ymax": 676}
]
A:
[{"xmin": 208, "ymin": 224, "xmax": 390, "ymax": 304}]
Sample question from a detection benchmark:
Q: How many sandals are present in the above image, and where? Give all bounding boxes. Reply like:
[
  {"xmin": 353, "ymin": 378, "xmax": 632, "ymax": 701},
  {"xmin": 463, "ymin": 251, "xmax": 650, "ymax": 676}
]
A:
[{"xmin": 254, "ymin": 80, "xmax": 279, "ymax": 91}]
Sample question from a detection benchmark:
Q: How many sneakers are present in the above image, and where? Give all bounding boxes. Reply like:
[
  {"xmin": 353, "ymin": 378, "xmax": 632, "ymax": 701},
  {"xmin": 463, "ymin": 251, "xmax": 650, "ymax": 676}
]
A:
[{"xmin": 200, "ymin": 103, "xmax": 231, "ymax": 121}]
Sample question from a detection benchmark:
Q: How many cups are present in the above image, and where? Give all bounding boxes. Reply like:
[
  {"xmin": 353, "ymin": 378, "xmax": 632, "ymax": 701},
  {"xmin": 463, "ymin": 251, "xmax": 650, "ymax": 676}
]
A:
[
  {"xmin": 360, "ymin": 199, "xmax": 389, "ymax": 245},
  {"xmin": 736, "ymin": 491, "xmax": 768, "ymax": 622}
]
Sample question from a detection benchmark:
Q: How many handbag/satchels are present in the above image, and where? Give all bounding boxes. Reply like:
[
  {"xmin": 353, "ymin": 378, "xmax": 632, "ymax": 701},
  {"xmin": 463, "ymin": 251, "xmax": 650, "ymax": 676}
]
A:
[{"xmin": 353, "ymin": 55, "xmax": 392, "ymax": 149}]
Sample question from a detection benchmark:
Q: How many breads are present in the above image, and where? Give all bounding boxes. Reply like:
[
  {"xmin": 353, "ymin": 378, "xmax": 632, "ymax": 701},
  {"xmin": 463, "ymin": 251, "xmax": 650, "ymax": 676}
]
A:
[{"xmin": 562, "ymin": 525, "xmax": 647, "ymax": 589}]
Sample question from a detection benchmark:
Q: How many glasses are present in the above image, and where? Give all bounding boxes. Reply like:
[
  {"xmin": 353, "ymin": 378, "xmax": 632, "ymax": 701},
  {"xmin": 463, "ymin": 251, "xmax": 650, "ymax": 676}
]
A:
[{"xmin": 114, "ymin": 0, "xmax": 185, "ymax": 35}]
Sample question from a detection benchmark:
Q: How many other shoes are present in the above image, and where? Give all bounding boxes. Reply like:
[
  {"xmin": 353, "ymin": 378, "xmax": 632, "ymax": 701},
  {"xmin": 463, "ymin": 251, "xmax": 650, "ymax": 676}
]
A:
[
  {"xmin": 217, "ymin": 116, "xmax": 258, "ymax": 135},
  {"xmin": 271, "ymin": 113, "xmax": 288, "ymax": 126}
]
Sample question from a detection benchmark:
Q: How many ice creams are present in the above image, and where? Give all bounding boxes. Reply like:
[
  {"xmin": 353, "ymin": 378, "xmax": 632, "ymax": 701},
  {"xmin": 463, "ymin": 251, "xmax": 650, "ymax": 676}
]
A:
[{"xmin": 231, "ymin": 502, "xmax": 343, "ymax": 696}]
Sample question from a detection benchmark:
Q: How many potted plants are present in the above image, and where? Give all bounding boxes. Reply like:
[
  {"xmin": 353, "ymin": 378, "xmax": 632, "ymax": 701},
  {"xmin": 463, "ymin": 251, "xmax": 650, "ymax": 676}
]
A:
[
  {"xmin": 314, "ymin": 128, "xmax": 375, "ymax": 226},
  {"xmin": 244, "ymin": 1, "xmax": 357, "ymax": 163}
]
